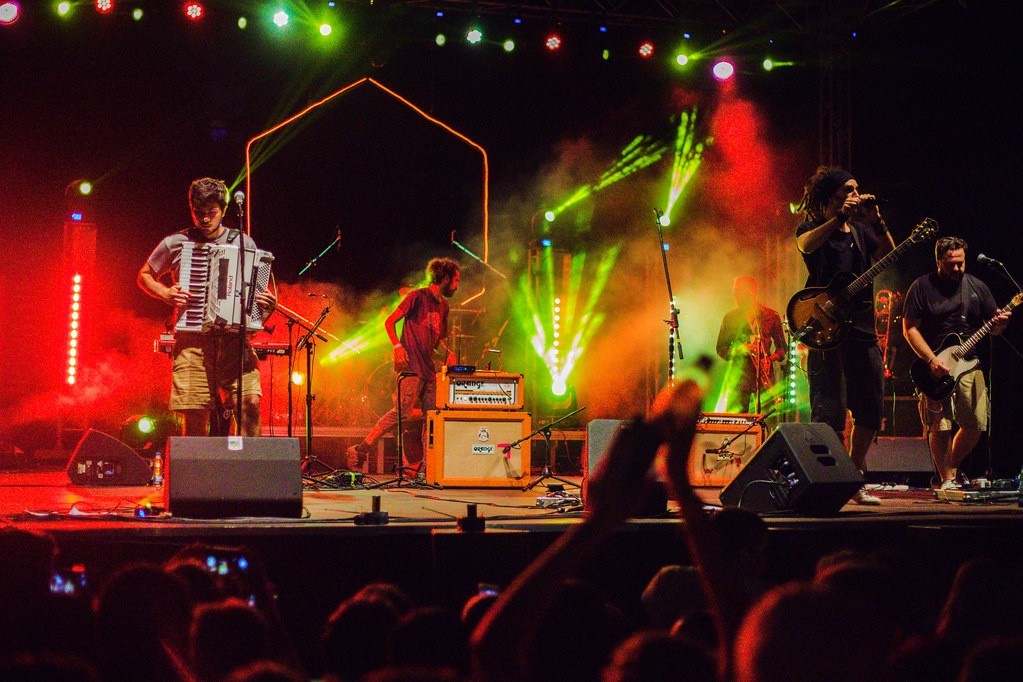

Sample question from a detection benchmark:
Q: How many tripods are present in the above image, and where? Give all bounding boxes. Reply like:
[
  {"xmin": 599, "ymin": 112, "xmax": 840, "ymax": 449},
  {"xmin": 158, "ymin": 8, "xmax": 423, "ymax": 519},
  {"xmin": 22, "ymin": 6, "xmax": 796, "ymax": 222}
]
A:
[
  {"xmin": 510, "ymin": 406, "xmax": 586, "ymax": 491},
  {"xmin": 295, "ymin": 306, "xmax": 336, "ymax": 478},
  {"xmin": 366, "ymin": 369, "xmax": 443, "ymax": 490}
]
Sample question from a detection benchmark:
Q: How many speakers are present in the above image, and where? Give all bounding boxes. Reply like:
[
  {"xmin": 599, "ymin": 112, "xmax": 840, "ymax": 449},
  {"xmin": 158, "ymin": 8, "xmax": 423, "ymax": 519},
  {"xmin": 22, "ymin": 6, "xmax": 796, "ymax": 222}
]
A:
[
  {"xmin": 67, "ymin": 428, "xmax": 153, "ymax": 487},
  {"xmin": 425, "ymin": 410, "xmax": 531, "ymax": 487},
  {"xmin": 688, "ymin": 411, "xmax": 940, "ymax": 516},
  {"xmin": 164, "ymin": 435, "xmax": 302, "ymax": 517},
  {"xmin": 582, "ymin": 419, "xmax": 630, "ymax": 501}
]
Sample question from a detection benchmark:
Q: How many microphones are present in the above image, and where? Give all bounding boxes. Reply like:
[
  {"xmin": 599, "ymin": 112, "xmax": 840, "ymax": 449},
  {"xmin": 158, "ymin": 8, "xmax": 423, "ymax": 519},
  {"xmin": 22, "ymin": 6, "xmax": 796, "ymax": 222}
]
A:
[
  {"xmin": 503, "ymin": 446, "xmax": 510, "ymax": 454},
  {"xmin": 234, "ymin": 191, "xmax": 245, "ymax": 204},
  {"xmin": 861, "ymin": 199, "xmax": 889, "ymax": 208},
  {"xmin": 977, "ymin": 254, "xmax": 1003, "ymax": 266},
  {"xmin": 705, "ymin": 449, "xmax": 729, "ymax": 453},
  {"xmin": 450, "ymin": 232, "xmax": 454, "ymax": 247},
  {"xmin": 338, "ymin": 228, "xmax": 341, "ymax": 247}
]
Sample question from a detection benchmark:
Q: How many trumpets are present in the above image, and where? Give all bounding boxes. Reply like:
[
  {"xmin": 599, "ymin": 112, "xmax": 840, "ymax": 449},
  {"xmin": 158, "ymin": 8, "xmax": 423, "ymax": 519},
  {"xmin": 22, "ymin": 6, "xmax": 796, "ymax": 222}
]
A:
[{"xmin": 743, "ymin": 341, "xmax": 784, "ymax": 406}]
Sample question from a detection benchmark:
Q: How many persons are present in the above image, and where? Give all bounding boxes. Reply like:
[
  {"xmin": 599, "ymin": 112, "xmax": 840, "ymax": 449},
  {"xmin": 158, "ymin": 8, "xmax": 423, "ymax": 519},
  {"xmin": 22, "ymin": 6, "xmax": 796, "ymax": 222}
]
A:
[
  {"xmin": 137, "ymin": 178, "xmax": 276, "ymax": 437},
  {"xmin": 902, "ymin": 237, "xmax": 1012, "ymax": 490},
  {"xmin": 796, "ymin": 166, "xmax": 895, "ymax": 506},
  {"xmin": 716, "ymin": 275, "xmax": 787, "ymax": 413},
  {"xmin": 0, "ymin": 400, "xmax": 1023, "ymax": 682},
  {"xmin": 346, "ymin": 258, "xmax": 462, "ymax": 477}
]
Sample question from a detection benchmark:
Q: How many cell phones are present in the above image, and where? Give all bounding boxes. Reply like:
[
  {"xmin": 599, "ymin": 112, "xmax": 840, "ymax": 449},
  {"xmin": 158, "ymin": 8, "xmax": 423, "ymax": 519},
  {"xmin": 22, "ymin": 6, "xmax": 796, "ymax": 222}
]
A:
[{"xmin": 200, "ymin": 547, "xmax": 252, "ymax": 581}]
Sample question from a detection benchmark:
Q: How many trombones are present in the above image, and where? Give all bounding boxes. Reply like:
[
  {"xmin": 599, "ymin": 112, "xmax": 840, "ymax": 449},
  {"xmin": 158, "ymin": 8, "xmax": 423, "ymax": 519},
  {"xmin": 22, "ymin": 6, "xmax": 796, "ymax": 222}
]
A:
[{"xmin": 875, "ymin": 290, "xmax": 893, "ymax": 377}]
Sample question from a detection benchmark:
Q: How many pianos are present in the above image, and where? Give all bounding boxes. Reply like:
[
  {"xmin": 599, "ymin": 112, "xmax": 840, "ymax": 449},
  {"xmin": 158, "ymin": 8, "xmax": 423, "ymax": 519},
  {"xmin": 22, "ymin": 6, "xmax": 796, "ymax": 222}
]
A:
[{"xmin": 154, "ymin": 335, "xmax": 292, "ymax": 361}]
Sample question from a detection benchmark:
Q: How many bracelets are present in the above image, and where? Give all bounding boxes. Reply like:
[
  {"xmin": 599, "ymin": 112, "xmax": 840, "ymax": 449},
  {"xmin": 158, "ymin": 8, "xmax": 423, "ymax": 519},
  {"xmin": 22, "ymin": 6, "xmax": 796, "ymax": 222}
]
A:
[
  {"xmin": 393, "ymin": 344, "xmax": 401, "ymax": 348},
  {"xmin": 927, "ymin": 355, "xmax": 935, "ymax": 363},
  {"xmin": 837, "ymin": 209, "xmax": 850, "ymax": 222}
]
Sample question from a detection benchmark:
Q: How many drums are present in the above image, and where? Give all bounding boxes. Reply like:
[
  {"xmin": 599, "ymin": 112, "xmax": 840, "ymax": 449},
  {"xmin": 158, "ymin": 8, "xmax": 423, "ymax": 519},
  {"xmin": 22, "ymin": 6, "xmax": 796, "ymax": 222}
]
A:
[{"xmin": 364, "ymin": 359, "xmax": 394, "ymax": 418}]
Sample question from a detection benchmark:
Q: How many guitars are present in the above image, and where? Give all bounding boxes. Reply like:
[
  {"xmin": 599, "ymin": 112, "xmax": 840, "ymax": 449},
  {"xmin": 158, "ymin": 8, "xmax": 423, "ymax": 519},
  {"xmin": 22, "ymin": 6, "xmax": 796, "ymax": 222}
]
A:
[
  {"xmin": 909, "ymin": 292, "xmax": 1023, "ymax": 402},
  {"xmin": 785, "ymin": 217, "xmax": 939, "ymax": 352}
]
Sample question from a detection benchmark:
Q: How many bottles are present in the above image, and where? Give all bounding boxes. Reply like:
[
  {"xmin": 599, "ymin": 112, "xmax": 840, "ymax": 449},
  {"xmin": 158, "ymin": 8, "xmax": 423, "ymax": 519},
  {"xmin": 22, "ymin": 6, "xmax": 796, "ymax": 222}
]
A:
[{"xmin": 152, "ymin": 452, "xmax": 164, "ymax": 488}]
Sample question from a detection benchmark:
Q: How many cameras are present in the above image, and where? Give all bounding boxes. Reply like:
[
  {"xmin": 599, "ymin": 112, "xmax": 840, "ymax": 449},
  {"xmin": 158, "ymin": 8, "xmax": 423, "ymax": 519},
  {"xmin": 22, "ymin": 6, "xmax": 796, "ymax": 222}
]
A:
[{"xmin": 50, "ymin": 567, "xmax": 87, "ymax": 593}]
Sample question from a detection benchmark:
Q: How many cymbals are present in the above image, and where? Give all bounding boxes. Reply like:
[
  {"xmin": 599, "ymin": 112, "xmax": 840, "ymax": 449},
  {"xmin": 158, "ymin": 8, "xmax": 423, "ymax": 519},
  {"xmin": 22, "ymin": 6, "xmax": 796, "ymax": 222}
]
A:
[
  {"xmin": 449, "ymin": 308, "xmax": 478, "ymax": 313},
  {"xmin": 449, "ymin": 334, "xmax": 474, "ymax": 338}
]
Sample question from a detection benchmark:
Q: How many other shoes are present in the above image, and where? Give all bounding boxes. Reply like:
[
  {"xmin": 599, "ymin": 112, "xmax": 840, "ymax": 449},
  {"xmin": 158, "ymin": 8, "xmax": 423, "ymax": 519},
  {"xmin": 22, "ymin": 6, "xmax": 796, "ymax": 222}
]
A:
[
  {"xmin": 951, "ymin": 478, "xmax": 962, "ymax": 488},
  {"xmin": 941, "ymin": 480, "xmax": 957, "ymax": 491},
  {"xmin": 852, "ymin": 460, "xmax": 867, "ymax": 477},
  {"xmin": 346, "ymin": 444, "xmax": 364, "ymax": 473},
  {"xmin": 852, "ymin": 485, "xmax": 882, "ymax": 505}
]
